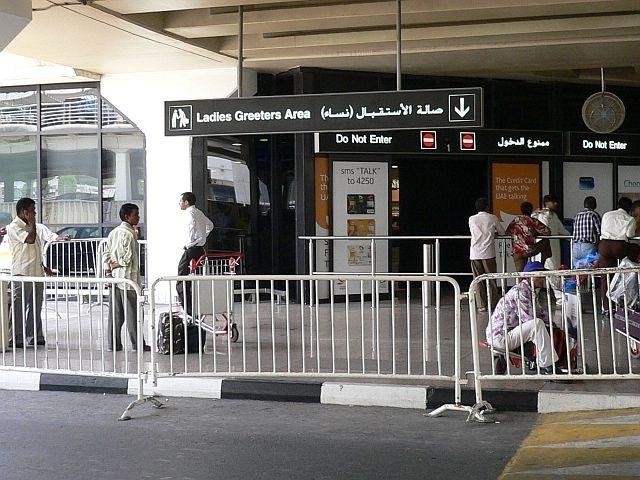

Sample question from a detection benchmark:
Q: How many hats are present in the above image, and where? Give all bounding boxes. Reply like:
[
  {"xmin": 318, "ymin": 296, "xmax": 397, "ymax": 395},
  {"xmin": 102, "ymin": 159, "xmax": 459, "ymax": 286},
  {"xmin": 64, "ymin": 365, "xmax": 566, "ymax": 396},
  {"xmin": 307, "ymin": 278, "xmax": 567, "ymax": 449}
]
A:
[
  {"xmin": 523, "ymin": 261, "xmax": 551, "ymax": 272},
  {"xmin": 537, "ymin": 288, "xmax": 555, "ymax": 299}
]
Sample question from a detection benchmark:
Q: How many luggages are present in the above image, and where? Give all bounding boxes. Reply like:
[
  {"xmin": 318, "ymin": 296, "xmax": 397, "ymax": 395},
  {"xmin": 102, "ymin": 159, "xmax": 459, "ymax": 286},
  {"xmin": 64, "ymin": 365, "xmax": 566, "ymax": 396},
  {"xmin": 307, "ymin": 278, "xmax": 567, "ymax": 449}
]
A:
[
  {"xmin": 157, "ymin": 309, "xmax": 206, "ymax": 355},
  {"xmin": 530, "ymin": 323, "xmax": 566, "ymax": 362}
]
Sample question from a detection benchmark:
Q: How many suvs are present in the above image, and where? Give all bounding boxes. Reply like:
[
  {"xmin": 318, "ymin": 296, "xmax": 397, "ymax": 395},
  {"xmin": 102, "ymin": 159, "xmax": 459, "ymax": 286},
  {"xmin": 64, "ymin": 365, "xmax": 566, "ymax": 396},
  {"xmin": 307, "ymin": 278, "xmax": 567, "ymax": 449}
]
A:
[{"xmin": 46, "ymin": 222, "xmax": 148, "ymax": 272}]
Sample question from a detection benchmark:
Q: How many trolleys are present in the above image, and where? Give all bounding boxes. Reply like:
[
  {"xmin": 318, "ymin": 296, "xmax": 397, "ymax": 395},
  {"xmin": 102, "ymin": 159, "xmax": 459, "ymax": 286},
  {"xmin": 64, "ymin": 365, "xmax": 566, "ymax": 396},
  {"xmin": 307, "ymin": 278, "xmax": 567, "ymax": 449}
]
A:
[
  {"xmin": 173, "ymin": 249, "xmax": 248, "ymax": 345},
  {"xmin": 604, "ymin": 259, "xmax": 640, "ymax": 359}
]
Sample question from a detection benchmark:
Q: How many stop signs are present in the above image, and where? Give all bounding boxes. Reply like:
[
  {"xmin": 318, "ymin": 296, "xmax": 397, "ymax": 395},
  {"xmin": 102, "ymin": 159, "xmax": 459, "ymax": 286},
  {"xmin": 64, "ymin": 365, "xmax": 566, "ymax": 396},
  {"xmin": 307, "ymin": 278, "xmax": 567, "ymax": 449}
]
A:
[
  {"xmin": 422, "ymin": 134, "xmax": 435, "ymax": 147},
  {"xmin": 462, "ymin": 134, "xmax": 474, "ymax": 148}
]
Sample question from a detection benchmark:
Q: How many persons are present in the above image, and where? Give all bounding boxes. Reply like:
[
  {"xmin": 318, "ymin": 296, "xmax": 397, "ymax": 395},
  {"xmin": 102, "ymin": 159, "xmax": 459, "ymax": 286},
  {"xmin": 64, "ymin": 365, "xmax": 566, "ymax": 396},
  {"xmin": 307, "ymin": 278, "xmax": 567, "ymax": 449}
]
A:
[
  {"xmin": 0, "ymin": 198, "xmax": 71, "ymax": 353},
  {"xmin": 176, "ymin": 193, "xmax": 214, "ymax": 316},
  {"xmin": 106, "ymin": 204, "xmax": 151, "ymax": 351},
  {"xmin": 468, "ymin": 194, "xmax": 640, "ymax": 383}
]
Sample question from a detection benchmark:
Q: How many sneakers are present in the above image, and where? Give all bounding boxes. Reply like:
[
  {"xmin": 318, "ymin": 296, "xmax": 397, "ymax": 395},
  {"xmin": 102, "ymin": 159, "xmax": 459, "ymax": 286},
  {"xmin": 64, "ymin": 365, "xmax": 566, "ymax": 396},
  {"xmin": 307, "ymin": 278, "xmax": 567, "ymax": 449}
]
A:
[
  {"xmin": 477, "ymin": 305, "xmax": 486, "ymax": 312},
  {"xmin": 108, "ymin": 344, "xmax": 124, "ymax": 352},
  {"xmin": 131, "ymin": 345, "xmax": 151, "ymax": 351},
  {"xmin": 27, "ymin": 338, "xmax": 46, "ymax": 346},
  {"xmin": 543, "ymin": 370, "xmax": 572, "ymax": 383},
  {"xmin": 8, "ymin": 340, "xmax": 24, "ymax": 349}
]
